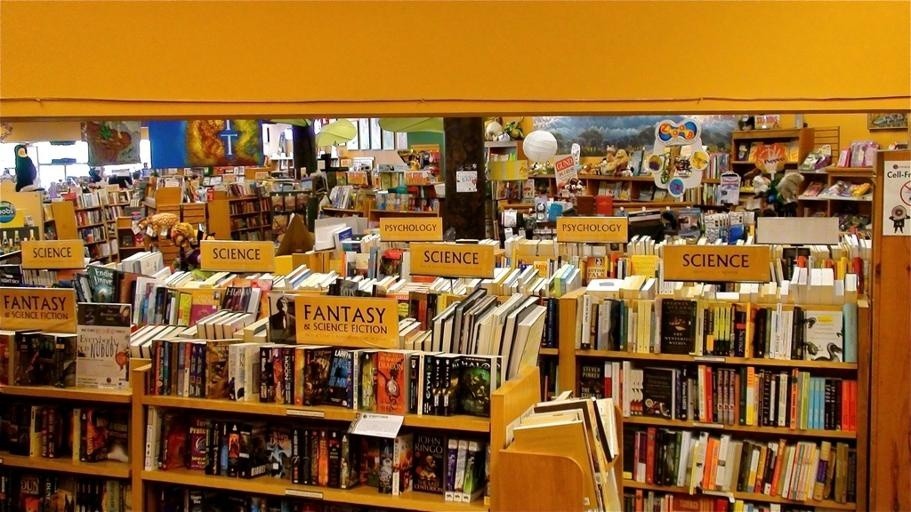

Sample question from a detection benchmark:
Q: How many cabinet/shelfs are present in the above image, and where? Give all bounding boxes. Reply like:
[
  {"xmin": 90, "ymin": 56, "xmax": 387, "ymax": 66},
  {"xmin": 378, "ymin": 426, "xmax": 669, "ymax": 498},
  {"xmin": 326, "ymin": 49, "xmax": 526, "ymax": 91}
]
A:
[
  {"xmin": 440, "ymin": 129, "xmax": 875, "ymax": 250},
  {"xmin": 3, "ymin": 164, "xmax": 442, "ymax": 265},
  {"xmin": 2, "ymin": 261, "xmax": 624, "ymax": 512},
  {"xmin": 292, "ymin": 238, "xmax": 871, "ymax": 512}
]
